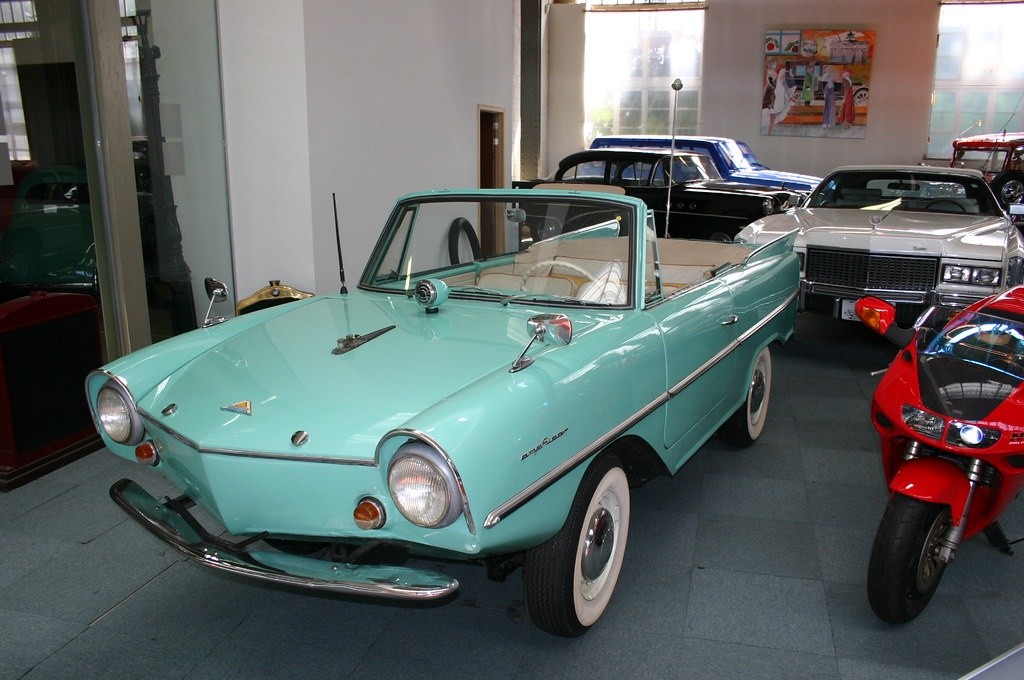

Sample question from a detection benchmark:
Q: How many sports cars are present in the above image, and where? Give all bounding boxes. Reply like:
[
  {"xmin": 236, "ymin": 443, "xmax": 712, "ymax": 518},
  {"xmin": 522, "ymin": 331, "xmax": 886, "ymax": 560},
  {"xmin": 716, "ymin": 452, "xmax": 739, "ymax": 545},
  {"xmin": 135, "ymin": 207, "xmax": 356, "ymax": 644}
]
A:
[{"xmin": 82, "ymin": 182, "xmax": 802, "ymax": 637}]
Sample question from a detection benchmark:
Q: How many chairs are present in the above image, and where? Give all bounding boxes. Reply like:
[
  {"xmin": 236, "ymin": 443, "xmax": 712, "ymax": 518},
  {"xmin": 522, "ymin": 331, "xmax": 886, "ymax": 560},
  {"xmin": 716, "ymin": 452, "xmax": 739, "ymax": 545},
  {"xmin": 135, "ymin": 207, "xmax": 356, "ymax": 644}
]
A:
[
  {"xmin": 478, "ymin": 274, "xmax": 573, "ymax": 298},
  {"xmin": 934, "ymin": 197, "xmax": 979, "ymax": 213},
  {"xmin": 837, "ymin": 188, "xmax": 881, "ymax": 206},
  {"xmin": 575, "ymin": 281, "xmax": 681, "ymax": 305}
]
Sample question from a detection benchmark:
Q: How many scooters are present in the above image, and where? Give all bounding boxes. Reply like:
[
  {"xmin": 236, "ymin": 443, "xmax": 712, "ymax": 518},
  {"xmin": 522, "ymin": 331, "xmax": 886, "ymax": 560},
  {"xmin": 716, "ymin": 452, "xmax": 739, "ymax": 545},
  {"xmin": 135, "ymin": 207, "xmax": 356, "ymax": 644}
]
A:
[{"xmin": 856, "ymin": 280, "xmax": 1024, "ymax": 628}]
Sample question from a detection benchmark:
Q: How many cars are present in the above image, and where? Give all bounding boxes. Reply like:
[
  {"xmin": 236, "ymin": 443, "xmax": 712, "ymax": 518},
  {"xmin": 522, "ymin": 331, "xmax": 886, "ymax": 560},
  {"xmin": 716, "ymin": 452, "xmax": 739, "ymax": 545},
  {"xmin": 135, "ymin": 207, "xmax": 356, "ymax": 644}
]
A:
[
  {"xmin": 1, "ymin": 157, "xmax": 160, "ymax": 301},
  {"xmin": 733, "ymin": 166, "xmax": 1024, "ymax": 332},
  {"xmin": 577, "ymin": 131, "xmax": 838, "ymax": 207},
  {"xmin": 917, "ymin": 130, "xmax": 1023, "ymax": 234},
  {"xmin": 510, "ymin": 147, "xmax": 803, "ymax": 244}
]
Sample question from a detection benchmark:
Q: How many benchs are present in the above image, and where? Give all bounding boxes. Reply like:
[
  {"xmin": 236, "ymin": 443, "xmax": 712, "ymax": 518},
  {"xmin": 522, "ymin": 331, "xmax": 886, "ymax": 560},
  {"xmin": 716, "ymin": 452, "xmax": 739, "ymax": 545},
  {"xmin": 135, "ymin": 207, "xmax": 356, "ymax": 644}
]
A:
[{"xmin": 515, "ymin": 238, "xmax": 753, "ymax": 284}]
